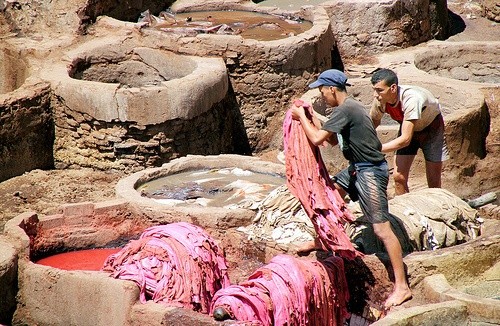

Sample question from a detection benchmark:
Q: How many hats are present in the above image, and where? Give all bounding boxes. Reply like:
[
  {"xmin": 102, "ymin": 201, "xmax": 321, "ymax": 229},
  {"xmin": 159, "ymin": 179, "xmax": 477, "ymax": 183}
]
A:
[{"xmin": 308, "ymin": 69, "xmax": 353, "ymax": 89}]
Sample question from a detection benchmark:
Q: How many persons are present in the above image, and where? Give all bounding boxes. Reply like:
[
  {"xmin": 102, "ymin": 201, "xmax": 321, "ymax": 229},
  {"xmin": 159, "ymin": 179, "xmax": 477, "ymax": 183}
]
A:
[
  {"xmin": 291, "ymin": 69, "xmax": 412, "ymax": 310},
  {"xmin": 370, "ymin": 68, "xmax": 445, "ymax": 197}
]
